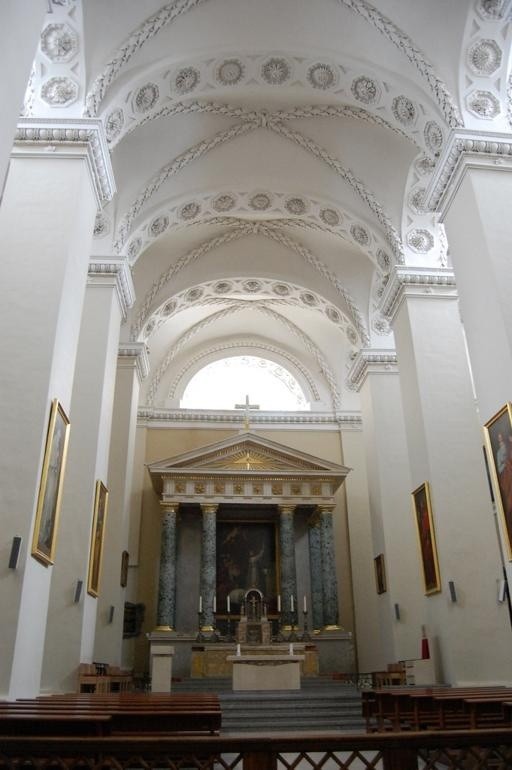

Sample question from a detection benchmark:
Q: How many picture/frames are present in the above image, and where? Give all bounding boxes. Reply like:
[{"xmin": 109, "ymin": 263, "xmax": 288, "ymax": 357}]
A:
[
  {"xmin": 373, "ymin": 554, "xmax": 385, "ymax": 594},
  {"xmin": 87, "ymin": 478, "xmax": 109, "ymax": 597},
  {"xmin": 31, "ymin": 398, "xmax": 71, "ymax": 565},
  {"xmin": 482, "ymin": 401, "xmax": 512, "ymax": 561},
  {"xmin": 410, "ymin": 482, "xmax": 441, "ymax": 598},
  {"xmin": 121, "ymin": 550, "xmax": 130, "ymax": 587}
]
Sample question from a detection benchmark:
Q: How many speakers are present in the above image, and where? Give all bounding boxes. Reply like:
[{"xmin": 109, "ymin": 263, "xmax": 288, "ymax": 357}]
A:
[
  {"xmin": 73, "ymin": 580, "xmax": 83, "ymax": 604},
  {"xmin": 448, "ymin": 581, "xmax": 457, "ymax": 603},
  {"xmin": 394, "ymin": 603, "xmax": 401, "ymax": 620},
  {"xmin": 108, "ymin": 606, "xmax": 114, "ymax": 623},
  {"xmin": 9, "ymin": 537, "xmax": 22, "ymax": 569}
]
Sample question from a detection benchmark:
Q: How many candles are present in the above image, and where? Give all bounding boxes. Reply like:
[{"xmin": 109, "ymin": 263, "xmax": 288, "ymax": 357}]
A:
[
  {"xmin": 291, "ymin": 595, "xmax": 294, "ymax": 611},
  {"xmin": 290, "ymin": 643, "xmax": 293, "ymax": 652},
  {"xmin": 236, "ymin": 644, "xmax": 240, "ymax": 652},
  {"xmin": 303, "ymin": 595, "xmax": 307, "ymax": 611},
  {"xmin": 227, "ymin": 595, "xmax": 230, "ymax": 611},
  {"xmin": 199, "ymin": 595, "xmax": 202, "ymax": 611},
  {"xmin": 278, "ymin": 595, "xmax": 281, "ymax": 610},
  {"xmin": 214, "ymin": 597, "xmax": 216, "ymax": 612}
]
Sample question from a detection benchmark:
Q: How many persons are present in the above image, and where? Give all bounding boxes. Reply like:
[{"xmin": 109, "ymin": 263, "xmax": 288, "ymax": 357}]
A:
[
  {"xmin": 247, "ymin": 543, "xmax": 272, "ymax": 593},
  {"xmin": 496, "ymin": 432, "xmax": 510, "ymax": 474}
]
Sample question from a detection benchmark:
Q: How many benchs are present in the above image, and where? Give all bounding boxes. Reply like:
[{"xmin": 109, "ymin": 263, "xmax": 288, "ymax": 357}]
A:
[
  {"xmin": 2, "ymin": 693, "xmax": 222, "ymax": 770},
  {"xmin": 362, "ymin": 687, "xmax": 512, "ymax": 769}
]
